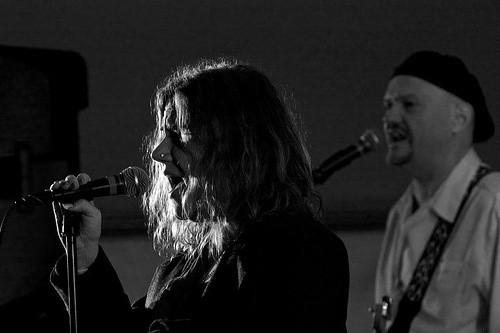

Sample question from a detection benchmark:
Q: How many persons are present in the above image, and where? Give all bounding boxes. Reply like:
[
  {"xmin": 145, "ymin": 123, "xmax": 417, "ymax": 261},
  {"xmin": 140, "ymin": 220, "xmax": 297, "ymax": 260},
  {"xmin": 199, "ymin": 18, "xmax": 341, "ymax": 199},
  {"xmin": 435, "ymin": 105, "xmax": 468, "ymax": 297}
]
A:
[
  {"xmin": 371, "ymin": 48, "xmax": 500, "ymax": 333},
  {"xmin": 48, "ymin": 59, "xmax": 350, "ymax": 333}
]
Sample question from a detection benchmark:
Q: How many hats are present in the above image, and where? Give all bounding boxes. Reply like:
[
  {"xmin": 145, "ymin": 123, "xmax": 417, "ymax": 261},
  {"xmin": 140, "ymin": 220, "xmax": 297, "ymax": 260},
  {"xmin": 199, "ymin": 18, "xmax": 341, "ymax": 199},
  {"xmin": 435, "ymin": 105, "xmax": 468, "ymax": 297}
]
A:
[{"xmin": 390, "ymin": 50, "xmax": 493, "ymax": 144}]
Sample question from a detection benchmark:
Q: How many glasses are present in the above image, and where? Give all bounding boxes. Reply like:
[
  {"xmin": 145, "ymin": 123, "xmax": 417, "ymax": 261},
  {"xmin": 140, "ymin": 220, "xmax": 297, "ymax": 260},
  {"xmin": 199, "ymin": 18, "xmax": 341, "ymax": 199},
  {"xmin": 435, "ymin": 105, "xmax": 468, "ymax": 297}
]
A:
[{"xmin": 160, "ymin": 125, "xmax": 198, "ymax": 149}]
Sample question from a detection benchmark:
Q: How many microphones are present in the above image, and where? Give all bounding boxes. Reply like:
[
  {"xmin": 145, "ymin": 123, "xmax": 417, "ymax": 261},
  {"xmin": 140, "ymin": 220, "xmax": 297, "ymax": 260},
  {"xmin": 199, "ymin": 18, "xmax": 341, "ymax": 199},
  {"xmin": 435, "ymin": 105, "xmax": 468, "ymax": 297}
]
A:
[
  {"xmin": 312, "ymin": 130, "xmax": 379, "ymax": 186},
  {"xmin": 22, "ymin": 167, "xmax": 150, "ymax": 204}
]
